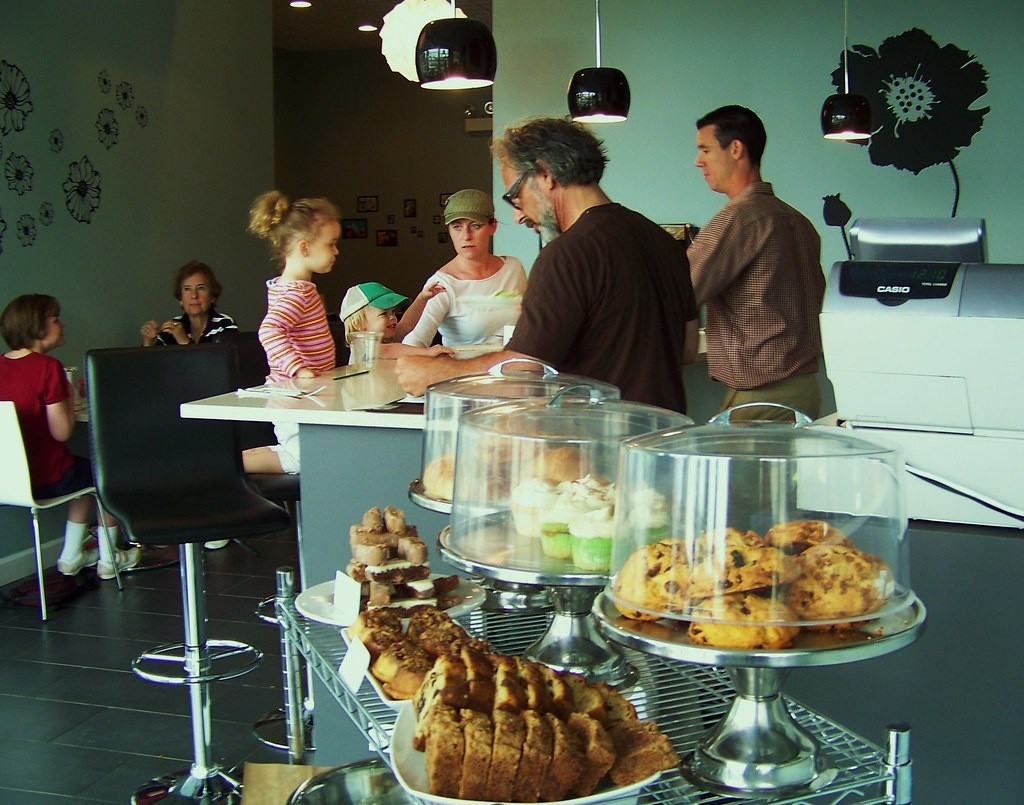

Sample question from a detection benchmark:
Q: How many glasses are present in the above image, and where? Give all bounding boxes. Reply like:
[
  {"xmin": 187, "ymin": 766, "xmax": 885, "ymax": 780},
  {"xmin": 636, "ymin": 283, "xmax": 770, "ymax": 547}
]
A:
[{"xmin": 502, "ymin": 172, "xmax": 534, "ymax": 210}]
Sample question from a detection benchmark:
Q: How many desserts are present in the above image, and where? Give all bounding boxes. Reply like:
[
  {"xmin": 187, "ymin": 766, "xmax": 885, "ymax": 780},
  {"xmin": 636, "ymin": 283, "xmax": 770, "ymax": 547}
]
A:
[{"xmin": 511, "ymin": 473, "xmax": 676, "ymax": 576}]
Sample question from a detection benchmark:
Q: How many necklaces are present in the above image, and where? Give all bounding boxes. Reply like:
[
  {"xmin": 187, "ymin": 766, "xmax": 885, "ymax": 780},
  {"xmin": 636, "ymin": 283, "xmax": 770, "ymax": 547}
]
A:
[{"xmin": 191, "ymin": 321, "xmax": 206, "ymax": 339}]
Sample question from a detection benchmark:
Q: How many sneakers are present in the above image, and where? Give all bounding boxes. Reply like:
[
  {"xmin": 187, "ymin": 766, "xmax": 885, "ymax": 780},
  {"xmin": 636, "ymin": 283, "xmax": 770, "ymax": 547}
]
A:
[
  {"xmin": 57, "ymin": 547, "xmax": 100, "ymax": 575},
  {"xmin": 98, "ymin": 547, "xmax": 141, "ymax": 578}
]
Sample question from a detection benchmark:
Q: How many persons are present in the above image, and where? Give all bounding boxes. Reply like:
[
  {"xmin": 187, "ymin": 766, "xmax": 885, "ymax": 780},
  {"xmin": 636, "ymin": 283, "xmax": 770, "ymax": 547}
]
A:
[
  {"xmin": 687, "ymin": 105, "xmax": 836, "ymax": 423},
  {"xmin": 141, "ymin": 260, "xmax": 241, "ymax": 549},
  {"xmin": 402, "ymin": 189, "xmax": 527, "ymax": 348},
  {"xmin": 0, "ymin": 293, "xmax": 142, "ymax": 579},
  {"xmin": 395, "ymin": 117, "xmax": 700, "ymax": 416},
  {"xmin": 241, "ymin": 190, "xmax": 341, "ymax": 475},
  {"xmin": 339, "ymin": 281, "xmax": 456, "ymax": 365}
]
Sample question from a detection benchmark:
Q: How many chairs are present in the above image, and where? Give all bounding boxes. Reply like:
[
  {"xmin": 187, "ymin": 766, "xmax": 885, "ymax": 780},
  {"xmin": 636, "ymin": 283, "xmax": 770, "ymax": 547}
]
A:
[
  {"xmin": 0, "ymin": 400, "xmax": 124, "ymax": 619},
  {"xmin": 83, "ymin": 343, "xmax": 292, "ymax": 805},
  {"xmin": 231, "ymin": 332, "xmax": 304, "ymax": 626}
]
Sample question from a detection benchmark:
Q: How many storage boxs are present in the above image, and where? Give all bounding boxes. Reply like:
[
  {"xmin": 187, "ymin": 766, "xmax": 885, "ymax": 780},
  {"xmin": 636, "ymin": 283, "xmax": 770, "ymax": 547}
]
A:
[{"xmin": 66, "ymin": 371, "xmax": 79, "ymax": 388}]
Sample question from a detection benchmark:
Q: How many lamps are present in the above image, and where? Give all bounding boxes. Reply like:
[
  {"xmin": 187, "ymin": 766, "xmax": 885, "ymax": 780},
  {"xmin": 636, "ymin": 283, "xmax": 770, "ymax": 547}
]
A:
[
  {"xmin": 567, "ymin": 0, "xmax": 632, "ymax": 125},
  {"xmin": 819, "ymin": 0, "xmax": 872, "ymax": 141},
  {"xmin": 415, "ymin": 0, "xmax": 499, "ymax": 92}
]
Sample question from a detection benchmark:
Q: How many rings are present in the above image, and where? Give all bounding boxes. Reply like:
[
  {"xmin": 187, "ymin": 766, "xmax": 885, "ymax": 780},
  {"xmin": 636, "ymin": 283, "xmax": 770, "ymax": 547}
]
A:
[{"xmin": 173, "ymin": 322, "xmax": 178, "ymax": 326}]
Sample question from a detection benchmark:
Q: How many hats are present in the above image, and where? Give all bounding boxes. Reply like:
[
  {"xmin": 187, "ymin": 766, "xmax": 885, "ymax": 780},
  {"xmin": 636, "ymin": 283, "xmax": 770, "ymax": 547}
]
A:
[
  {"xmin": 340, "ymin": 282, "xmax": 411, "ymax": 323},
  {"xmin": 444, "ymin": 190, "xmax": 495, "ymax": 226}
]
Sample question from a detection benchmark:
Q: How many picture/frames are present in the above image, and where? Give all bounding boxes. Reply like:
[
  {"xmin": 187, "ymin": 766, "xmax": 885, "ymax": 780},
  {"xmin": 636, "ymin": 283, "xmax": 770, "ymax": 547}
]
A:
[
  {"xmin": 376, "ymin": 229, "xmax": 398, "ymax": 247},
  {"xmin": 357, "ymin": 196, "xmax": 378, "ymax": 213},
  {"xmin": 411, "ymin": 226, "xmax": 416, "ymax": 233},
  {"xmin": 342, "ymin": 218, "xmax": 368, "ymax": 240},
  {"xmin": 387, "ymin": 215, "xmax": 395, "ymax": 224},
  {"xmin": 403, "ymin": 198, "xmax": 417, "ymax": 218},
  {"xmin": 433, "ymin": 215, "xmax": 441, "ymax": 225},
  {"xmin": 438, "ymin": 232, "xmax": 449, "ymax": 243},
  {"xmin": 418, "ymin": 230, "xmax": 424, "ymax": 237},
  {"xmin": 440, "ymin": 192, "xmax": 455, "ymax": 207}
]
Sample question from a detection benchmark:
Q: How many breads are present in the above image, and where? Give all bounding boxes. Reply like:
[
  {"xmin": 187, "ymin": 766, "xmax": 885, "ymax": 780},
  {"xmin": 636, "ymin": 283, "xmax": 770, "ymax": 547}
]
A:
[
  {"xmin": 349, "ymin": 604, "xmax": 684, "ymax": 805},
  {"xmin": 612, "ymin": 520, "xmax": 898, "ymax": 651},
  {"xmin": 344, "ymin": 505, "xmax": 464, "ymax": 618},
  {"xmin": 425, "ymin": 422, "xmax": 597, "ymax": 506}
]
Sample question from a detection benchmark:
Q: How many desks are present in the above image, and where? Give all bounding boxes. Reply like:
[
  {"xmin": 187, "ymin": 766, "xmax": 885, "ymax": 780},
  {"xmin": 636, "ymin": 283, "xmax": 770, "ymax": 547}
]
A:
[{"xmin": 72, "ymin": 398, "xmax": 89, "ymax": 423}]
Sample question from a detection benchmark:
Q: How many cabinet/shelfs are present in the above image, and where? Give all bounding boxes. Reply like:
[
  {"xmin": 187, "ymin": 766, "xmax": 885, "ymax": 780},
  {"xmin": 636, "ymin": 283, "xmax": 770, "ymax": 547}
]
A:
[{"xmin": 270, "ymin": 565, "xmax": 913, "ymax": 805}]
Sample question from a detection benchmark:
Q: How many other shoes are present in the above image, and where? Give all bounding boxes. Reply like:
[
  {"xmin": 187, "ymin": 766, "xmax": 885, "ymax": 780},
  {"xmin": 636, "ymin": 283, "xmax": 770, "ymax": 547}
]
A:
[{"xmin": 205, "ymin": 539, "xmax": 229, "ymax": 549}]
